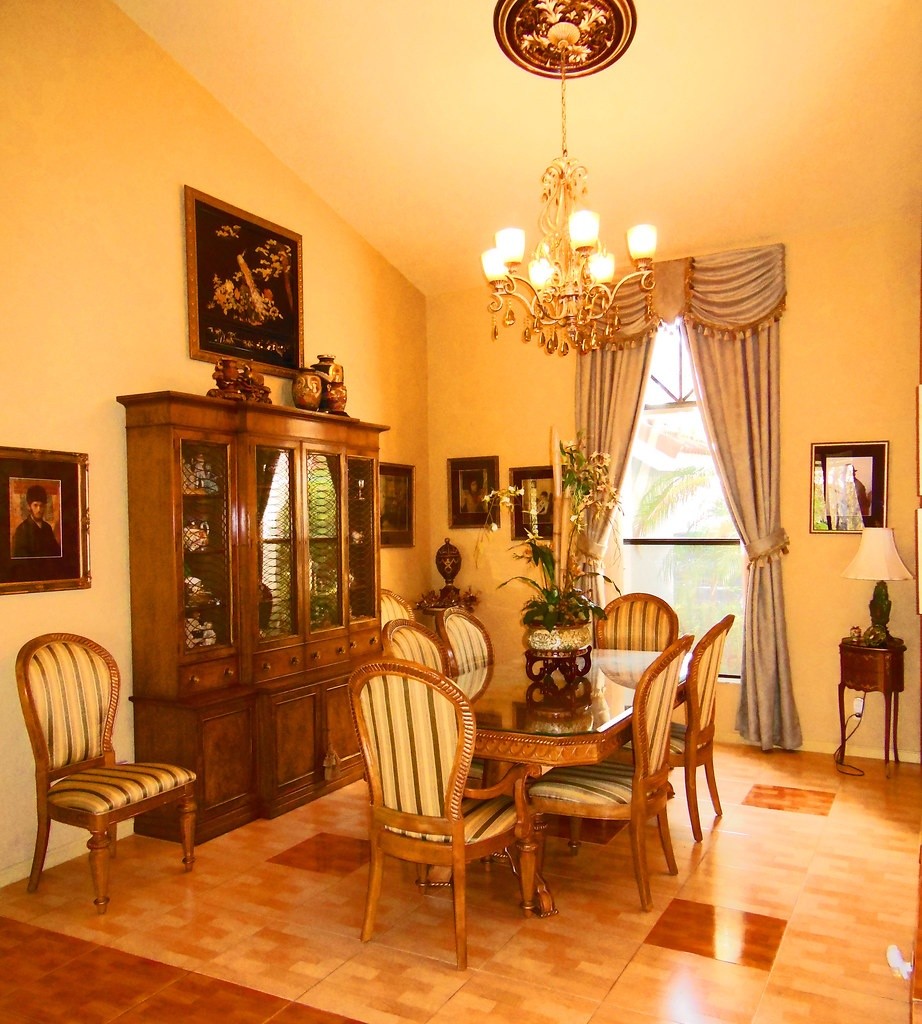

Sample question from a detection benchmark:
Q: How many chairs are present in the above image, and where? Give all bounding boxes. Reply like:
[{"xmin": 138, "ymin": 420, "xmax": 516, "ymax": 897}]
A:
[
  {"xmin": 15, "ymin": 632, "xmax": 199, "ymax": 915},
  {"xmin": 348, "ymin": 590, "xmax": 734, "ymax": 970}
]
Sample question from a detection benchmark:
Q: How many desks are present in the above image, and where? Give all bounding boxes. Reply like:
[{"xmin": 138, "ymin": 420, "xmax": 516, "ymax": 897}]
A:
[
  {"xmin": 424, "ymin": 606, "xmax": 464, "ymax": 632},
  {"xmin": 446, "ymin": 649, "xmax": 663, "ymax": 917},
  {"xmin": 838, "ymin": 637, "xmax": 907, "ymax": 780}
]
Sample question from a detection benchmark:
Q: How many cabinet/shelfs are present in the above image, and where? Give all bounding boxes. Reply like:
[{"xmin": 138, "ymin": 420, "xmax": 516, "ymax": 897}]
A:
[{"xmin": 118, "ymin": 392, "xmax": 392, "ymax": 846}]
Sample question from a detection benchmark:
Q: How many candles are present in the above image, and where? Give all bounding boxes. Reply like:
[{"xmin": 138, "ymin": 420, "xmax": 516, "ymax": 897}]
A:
[{"xmin": 530, "ymin": 427, "xmax": 571, "ymax": 586}]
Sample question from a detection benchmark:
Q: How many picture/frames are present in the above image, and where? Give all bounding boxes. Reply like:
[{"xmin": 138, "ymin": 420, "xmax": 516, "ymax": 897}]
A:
[
  {"xmin": 509, "ymin": 466, "xmax": 567, "ymax": 541},
  {"xmin": 809, "ymin": 440, "xmax": 890, "ymax": 534},
  {"xmin": 446, "ymin": 457, "xmax": 500, "ymax": 529},
  {"xmin": 0, "ymin": 446, "xmax": 93, "ymax": 597},
  {"xmin": 184, "ymin": 185, "xmax": 304, "ymax": 379},
  {"xmin": 378, "ymin": 462, "xmax": 416, "ymax": 548}
]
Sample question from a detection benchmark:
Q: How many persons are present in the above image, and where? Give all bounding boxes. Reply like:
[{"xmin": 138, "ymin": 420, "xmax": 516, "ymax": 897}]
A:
[
  {"xmin": 845, "ymin": 466, "xmax": 870, "ymax": 515},
  {"xmin": 11, "ymin": 486, "xmax": 60, "ymax": 557}
]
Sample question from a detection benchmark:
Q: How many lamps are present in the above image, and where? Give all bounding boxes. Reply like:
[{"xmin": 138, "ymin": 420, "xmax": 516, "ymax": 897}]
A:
[
  {"xmin": 843, "ymin": 528, "xmax": 915, "ymax": 646},
  {"xmin": 481, "ymin": 0, "xmax": 659, "ymax": 358}
]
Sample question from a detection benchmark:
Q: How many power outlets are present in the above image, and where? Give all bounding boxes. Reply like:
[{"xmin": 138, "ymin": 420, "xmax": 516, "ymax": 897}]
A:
[{"xmin": 853, "ymin": 698, "xmax": 864, "ymax": 720}]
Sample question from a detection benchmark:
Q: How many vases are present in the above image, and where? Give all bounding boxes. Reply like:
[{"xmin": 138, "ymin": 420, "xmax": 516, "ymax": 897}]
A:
[
  {"xmin": 326, "ymin": 382, "xmax": 347, "ymax": 414},
  {"xmin": 312, "ymin": 355, "xmax": 344, "ymax": 410},
  {"xmin": 291, "ymin": 368, "xmax": 322, "ymax": 410},
  {"xmin": 524, "ymin": 621, "xmax": 593, "ymax": 653}
]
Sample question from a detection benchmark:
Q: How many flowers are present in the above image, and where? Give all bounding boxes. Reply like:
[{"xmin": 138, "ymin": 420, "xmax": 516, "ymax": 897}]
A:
[{"xmin": 471, "ymin": 430, "xmax": 625, "ymax": 633}]
